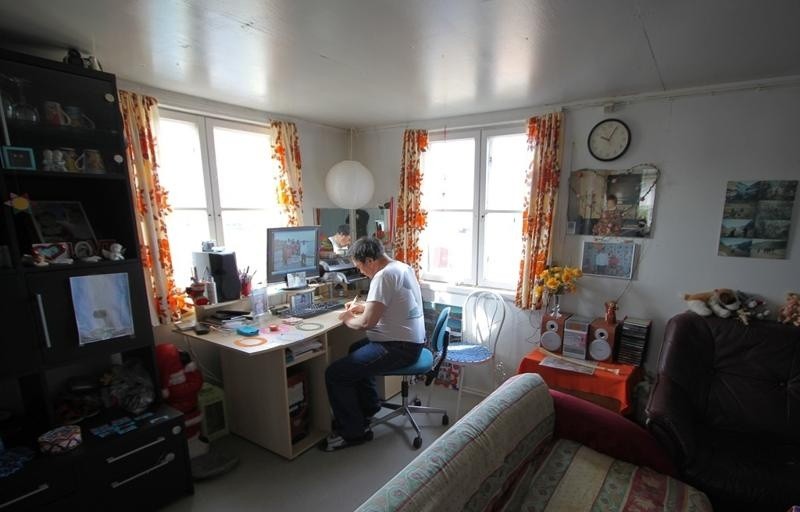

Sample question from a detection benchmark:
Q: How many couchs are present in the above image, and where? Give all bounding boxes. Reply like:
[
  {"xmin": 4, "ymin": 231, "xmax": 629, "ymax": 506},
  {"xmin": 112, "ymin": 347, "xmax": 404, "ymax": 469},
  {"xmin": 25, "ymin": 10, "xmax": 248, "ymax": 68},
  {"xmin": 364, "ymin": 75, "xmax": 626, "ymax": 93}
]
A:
[{"xmin": 353, "ymin": 373, "xmax": 713, "ymax": 512}]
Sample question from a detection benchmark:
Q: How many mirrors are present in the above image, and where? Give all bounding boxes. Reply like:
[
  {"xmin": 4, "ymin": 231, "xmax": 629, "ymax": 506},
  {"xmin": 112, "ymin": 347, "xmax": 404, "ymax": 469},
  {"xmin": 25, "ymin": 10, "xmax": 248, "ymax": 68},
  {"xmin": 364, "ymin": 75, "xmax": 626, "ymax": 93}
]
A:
[{"xmin": 313, "ymin": 208, "xmax": 391, "ymax": 258}]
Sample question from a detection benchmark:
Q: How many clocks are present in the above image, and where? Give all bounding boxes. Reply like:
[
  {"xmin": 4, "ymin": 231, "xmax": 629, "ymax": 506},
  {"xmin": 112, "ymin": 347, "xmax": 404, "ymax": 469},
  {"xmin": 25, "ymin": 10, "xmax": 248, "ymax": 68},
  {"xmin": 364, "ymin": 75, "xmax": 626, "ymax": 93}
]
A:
[{"xmin": 587, "ymin": 118, "xmax": 631, "ymax": 161}]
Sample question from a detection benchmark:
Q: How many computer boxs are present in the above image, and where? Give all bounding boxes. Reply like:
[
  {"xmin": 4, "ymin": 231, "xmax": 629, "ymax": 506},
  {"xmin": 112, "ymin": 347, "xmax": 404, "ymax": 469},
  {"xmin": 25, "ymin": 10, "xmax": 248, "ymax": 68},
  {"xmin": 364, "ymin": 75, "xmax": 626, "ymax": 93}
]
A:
[{"xmin": 287, "ymin": 367, "xmax": 308, "ymax": 445}]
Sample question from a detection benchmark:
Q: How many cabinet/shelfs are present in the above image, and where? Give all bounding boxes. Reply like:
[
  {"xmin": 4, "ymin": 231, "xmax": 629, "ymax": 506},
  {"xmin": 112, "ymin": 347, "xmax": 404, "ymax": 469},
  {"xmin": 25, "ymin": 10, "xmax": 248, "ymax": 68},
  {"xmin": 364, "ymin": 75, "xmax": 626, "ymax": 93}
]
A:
[
  {"xmin": 0, "ymin": 436, "xmax": 88, "ymax": 512},
  {"xmin": 81, "ymin": 402, "xmax": 195, "ymax": 512},
  {"xmin": 1, "ymin": 49, "xmax": 161, "ymax": 421}
]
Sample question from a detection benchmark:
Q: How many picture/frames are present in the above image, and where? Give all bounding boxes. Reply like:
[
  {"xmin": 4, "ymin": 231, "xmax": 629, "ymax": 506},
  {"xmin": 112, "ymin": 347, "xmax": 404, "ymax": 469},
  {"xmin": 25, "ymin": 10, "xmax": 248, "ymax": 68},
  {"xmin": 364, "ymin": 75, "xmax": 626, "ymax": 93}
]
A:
[
  {"xmin": 3, "ymin": 147, "xmax": 37, "ymax": 171},
  {"xmin": 580, "ymin": 240, "xmax": 636, "ymax": 279},
  {"xmin": 28, "ymin": 200, "xmax": 97, "ymax": 244}
]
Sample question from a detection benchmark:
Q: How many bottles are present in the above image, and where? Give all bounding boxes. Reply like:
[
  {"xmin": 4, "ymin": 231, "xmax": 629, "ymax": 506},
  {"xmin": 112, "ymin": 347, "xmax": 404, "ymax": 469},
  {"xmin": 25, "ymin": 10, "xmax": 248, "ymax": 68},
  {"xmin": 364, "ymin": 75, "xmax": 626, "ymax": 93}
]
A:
[{"xmin": 205, "ymin": 275, "xmax": 218, "ymax": 304}]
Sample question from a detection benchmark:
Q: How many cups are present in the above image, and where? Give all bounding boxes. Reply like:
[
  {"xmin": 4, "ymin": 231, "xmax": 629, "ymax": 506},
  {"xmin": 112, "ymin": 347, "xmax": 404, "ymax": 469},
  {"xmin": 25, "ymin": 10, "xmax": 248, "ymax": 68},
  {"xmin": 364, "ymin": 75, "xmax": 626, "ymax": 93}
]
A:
[
  {"xmin": 45, "ymin": 99, "xmax": 71, "ymax": 125},
  {"xmin": 239, "ymin": 279, "xmax": 252, "ymax": 299},
  {"xmin": 65, "ymin": 106, "xmax": 96, "ymax": 130},
  {"xmin": 62, "ymin": 147, "xmax": 81, "ymax": 173},
  {"xmin": 74, "ymin": 150, "xmax": 105, "ymax": 175}
]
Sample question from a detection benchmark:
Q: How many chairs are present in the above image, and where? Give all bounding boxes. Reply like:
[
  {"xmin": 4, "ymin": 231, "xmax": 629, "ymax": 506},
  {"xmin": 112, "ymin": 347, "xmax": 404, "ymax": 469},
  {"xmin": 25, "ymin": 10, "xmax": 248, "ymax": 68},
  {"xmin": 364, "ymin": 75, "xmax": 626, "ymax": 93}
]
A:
[
  {"xmin": 426, "ymin": 290, "xmax": 506, "ymax": 421},
  {"xmin": 364, "ymin": 306, "xmax": 451, "ymax": 449},
  {"xmin": 643, "ymin": 310, "xmax": 800, "ymax": 512}
]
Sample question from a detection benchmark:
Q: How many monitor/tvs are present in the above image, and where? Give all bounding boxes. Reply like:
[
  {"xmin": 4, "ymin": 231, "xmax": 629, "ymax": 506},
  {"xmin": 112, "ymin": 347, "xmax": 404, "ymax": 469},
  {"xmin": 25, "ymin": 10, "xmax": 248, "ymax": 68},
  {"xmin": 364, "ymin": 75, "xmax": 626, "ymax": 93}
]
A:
[{"xmin": 267, "ymin": 226, "xmax": 319, "ymax": 290}]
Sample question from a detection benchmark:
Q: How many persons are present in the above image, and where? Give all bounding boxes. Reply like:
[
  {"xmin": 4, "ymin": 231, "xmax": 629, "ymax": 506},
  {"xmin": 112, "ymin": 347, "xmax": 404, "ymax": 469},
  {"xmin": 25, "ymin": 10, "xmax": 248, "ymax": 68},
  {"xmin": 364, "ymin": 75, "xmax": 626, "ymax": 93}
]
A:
[
  {"xmin": 280, "ymin": 237, "xmax": 307, "ymax": 266},
  {"xmin": 317, "ymin": 233, "xmax": 430, "ymax": 454},
  {"xmin": 596, "ymin": 248, "xmax": 609, "ymax": 277},
  {"xmin": 591, "ymin": 195, "xmax": 624, "ymax": 238}
]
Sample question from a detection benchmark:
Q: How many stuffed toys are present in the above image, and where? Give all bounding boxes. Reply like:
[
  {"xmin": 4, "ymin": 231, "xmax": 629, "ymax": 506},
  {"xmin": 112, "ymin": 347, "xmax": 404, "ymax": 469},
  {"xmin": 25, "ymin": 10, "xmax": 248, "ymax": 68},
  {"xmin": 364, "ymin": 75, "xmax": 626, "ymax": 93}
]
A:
[
  {"xmin": 775, "ymin": 290, "xmax": 800, "ymax": 328},
  {"xmin": 679, "ymin": 287, "xmax": 742, "ymax": 319},
  {"xmin": 737, "ymin": 290, "xmax": 772, "ymax": 324},
  {"xmin": 42, "ymin": 147, "xmax": 68, "ymax": 173},
  {"xmin": 156, "ymin": 341, "xmax": 212, "ymax": 462}
]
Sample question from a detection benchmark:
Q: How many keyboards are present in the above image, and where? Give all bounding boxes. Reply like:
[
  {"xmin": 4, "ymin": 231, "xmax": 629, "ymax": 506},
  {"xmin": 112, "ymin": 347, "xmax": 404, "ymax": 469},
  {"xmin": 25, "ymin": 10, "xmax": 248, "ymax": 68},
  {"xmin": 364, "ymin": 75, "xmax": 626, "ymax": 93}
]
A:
[{"xmin": 292, "ymin": 300, "xmax": 343, "ymax": 318}]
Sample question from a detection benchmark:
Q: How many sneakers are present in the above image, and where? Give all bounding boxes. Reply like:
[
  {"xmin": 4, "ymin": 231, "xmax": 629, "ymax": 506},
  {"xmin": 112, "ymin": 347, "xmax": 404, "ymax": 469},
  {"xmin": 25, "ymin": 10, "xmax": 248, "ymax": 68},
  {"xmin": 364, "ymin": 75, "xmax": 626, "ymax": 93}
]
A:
[{"xmin": 322, "ymin": 426, "xmax": 374, "ymax": 452}]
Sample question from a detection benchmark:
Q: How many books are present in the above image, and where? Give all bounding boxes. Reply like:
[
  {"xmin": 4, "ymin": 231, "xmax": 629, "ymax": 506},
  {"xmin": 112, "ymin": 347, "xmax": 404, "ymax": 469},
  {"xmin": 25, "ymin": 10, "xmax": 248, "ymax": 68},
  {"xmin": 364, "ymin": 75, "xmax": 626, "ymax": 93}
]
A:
[{"xmin": 616, "ymin": 315, "xmax": 653, "ymax": 370}]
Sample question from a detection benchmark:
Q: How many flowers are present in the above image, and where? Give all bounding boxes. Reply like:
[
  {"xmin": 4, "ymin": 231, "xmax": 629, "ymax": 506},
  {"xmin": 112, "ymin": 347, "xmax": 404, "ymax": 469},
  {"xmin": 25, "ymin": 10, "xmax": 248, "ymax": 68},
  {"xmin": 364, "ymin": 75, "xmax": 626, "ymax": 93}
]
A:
[{"xmin": 534, "ymin": 265, "xmax": 583, "ymax": 297}]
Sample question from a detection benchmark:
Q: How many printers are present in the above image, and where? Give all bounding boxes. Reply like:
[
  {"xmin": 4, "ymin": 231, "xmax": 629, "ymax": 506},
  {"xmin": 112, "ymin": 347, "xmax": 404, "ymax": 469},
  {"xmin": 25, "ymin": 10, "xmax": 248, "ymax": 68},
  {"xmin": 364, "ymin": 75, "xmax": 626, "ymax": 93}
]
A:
[{"xmin": 319, "ymin": 258, "xmax": 368, "ymax": 284}]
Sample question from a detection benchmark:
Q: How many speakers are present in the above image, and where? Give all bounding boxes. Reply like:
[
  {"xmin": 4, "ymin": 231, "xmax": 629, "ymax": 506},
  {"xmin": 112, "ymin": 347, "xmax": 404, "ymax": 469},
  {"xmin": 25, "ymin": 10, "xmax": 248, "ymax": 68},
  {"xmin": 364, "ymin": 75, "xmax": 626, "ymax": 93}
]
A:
[
  {"xmin": 586, "ymin": 318, "xmax": 622, "ymax": 364},
  {"xmin": 192, "ymin": 249, "xmax": 239, "ymax": 303},
  {"xmin": 540, "ymin": 310, "xmax": 572, "ymax": 355}
]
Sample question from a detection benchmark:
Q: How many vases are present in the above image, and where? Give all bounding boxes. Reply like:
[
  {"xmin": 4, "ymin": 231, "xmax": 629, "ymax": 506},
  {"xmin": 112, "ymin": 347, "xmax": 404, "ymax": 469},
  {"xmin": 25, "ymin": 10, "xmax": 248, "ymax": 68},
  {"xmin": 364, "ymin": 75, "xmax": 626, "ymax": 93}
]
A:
[{"xmin": 550, "ymin": 294, "xmax": 562, "ymax": 318}]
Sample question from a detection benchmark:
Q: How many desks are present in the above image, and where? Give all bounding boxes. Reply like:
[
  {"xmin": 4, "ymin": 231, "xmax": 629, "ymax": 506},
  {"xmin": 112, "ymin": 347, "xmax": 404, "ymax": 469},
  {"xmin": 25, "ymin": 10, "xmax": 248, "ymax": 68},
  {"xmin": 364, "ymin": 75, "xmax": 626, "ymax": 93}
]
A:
[
  {"xmin": 171, "ymin": 274, "xmax": 407, "ymax": 460},
  {"xmin": 518, "ymin": 346, "xmax": 644, "ymax": 417}
]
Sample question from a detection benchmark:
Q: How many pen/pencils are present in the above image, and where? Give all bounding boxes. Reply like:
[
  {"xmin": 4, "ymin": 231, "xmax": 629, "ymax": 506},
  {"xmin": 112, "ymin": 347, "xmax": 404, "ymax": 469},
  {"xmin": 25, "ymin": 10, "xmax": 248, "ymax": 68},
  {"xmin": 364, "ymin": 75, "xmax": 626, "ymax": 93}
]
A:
[
  {"xmin": 347, "ymin": 294, "xmax": 359, "ymax": 311},
  {"xmin": 239, "ymin": 266, "xmax": 257, "ymax": 283},
  {"xmin": 191, "ymin": 266, "xmax": 209, "ymax": 284}
]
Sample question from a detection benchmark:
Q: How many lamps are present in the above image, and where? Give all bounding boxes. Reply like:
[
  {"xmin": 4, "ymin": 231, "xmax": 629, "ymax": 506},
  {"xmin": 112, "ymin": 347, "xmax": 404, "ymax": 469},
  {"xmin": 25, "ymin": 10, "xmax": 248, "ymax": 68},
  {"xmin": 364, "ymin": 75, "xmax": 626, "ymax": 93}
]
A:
[{"xmin": 325, "ymin": 128, "xmax": 374, "ymax": 209}]
